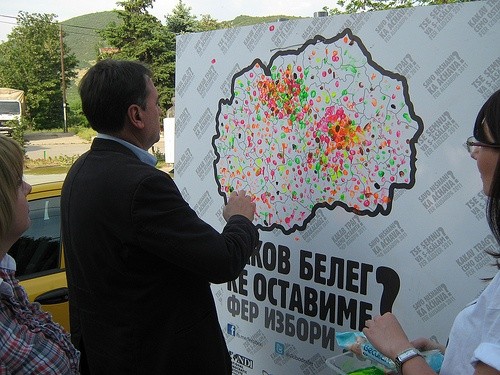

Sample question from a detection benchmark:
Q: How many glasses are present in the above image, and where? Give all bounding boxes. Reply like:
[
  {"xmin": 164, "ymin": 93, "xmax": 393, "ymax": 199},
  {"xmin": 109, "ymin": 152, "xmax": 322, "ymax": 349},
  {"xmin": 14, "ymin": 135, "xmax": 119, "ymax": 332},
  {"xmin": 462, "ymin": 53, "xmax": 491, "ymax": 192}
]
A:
[{"xmin": 466, "ymin": 135, "xmax": 499, "ymax": 154}]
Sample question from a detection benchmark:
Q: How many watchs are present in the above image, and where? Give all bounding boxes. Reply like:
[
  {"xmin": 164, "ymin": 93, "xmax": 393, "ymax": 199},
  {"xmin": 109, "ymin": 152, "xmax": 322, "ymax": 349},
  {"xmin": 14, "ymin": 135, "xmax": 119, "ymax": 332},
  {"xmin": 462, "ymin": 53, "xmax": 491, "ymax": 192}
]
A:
[{"xmin": 395, "ymin": 348, "xmax": 426, "ymax": 375}]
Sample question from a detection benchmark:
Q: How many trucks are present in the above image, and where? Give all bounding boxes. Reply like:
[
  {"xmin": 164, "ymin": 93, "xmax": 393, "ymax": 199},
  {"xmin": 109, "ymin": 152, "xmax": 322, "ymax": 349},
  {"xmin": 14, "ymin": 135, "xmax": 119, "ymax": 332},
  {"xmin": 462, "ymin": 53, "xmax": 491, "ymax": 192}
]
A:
[{"xmin": 0, "ymin": 87, "xmax": 25, "ymax": 140}]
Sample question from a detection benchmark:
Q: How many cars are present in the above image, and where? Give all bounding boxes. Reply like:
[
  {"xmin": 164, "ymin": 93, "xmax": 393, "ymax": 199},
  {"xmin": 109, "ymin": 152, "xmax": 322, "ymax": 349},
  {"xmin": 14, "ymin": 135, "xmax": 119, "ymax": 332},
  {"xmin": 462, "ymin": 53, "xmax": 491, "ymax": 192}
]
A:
[{"xmin": 5, "ymin": 164, "xmax": 174, "ymax": 335}]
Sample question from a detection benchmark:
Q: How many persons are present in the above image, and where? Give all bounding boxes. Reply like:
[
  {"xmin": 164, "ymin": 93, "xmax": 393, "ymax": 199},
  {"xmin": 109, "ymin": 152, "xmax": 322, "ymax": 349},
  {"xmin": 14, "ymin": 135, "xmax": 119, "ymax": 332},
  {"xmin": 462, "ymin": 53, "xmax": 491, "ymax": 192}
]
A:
[
  {"xmin": 60, "ymin": 59, "xmax": 259, "ymax": 375},
  {"xmin": 0, "ymin": 133, "xmax": 80, "ymax": 375},
  {"xmin": 363, "ymin": 90, "xmax": 500, "ymax": 375}
]
